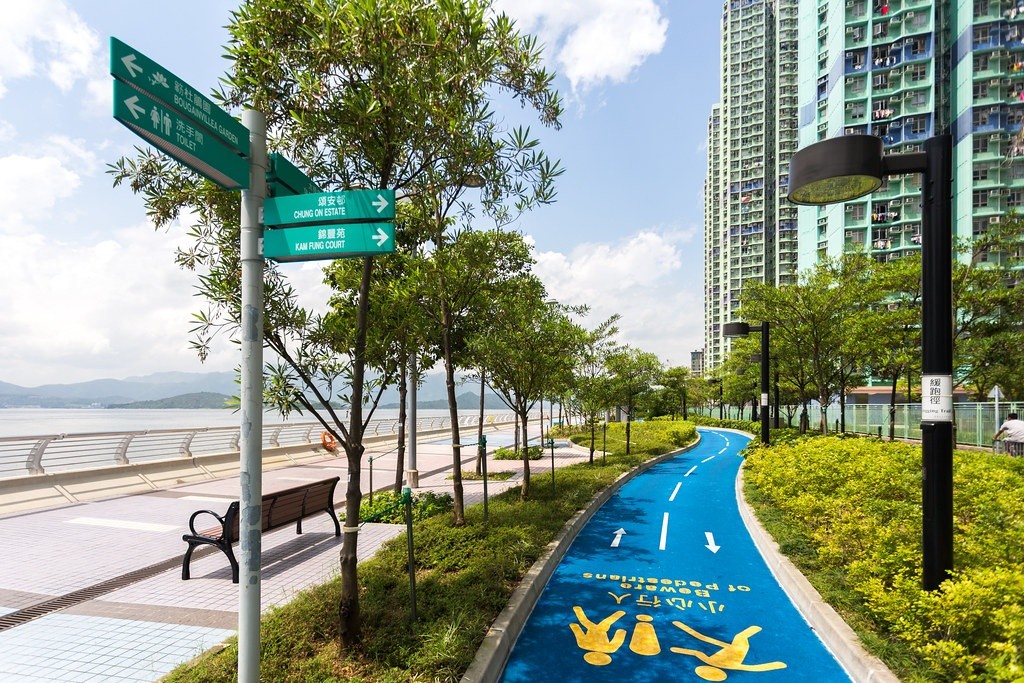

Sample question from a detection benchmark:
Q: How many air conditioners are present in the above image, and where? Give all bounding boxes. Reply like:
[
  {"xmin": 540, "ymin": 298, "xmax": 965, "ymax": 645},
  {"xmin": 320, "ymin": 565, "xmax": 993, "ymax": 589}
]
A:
[{"xmin": 817, "ymin": 0, "xmax": 1024, "ymax": 312}]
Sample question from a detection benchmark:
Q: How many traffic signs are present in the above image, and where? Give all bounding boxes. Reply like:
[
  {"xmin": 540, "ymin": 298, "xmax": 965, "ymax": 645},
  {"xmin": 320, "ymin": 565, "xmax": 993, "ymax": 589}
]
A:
[{"xmin": 108, "ymin": 34, "xmax": 397, "ymax": 263}]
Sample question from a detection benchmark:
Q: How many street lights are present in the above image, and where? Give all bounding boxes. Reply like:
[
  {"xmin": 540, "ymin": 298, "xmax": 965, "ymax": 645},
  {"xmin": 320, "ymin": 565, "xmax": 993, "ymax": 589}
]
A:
[
  {"xmin": 788, "ymin": 129, "xmax": 955, "ymax": 593},
  {"xmin": 723, "ymin": 321, "xmax": 771, "ymax": 451}
]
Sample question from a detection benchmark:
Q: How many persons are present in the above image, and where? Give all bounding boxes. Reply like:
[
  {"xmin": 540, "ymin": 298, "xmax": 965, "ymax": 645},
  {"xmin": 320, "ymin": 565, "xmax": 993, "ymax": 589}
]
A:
[{"xmin": 991, "ymin": 412, "xmax": 1024, "ymax": 456}]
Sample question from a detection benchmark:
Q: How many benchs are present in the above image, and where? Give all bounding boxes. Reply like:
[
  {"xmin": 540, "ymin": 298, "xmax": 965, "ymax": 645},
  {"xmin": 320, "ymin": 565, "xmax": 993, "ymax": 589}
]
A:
[{"xmin": 181, "ymin": 476, "xmax": 341, "ymax": 583}]
